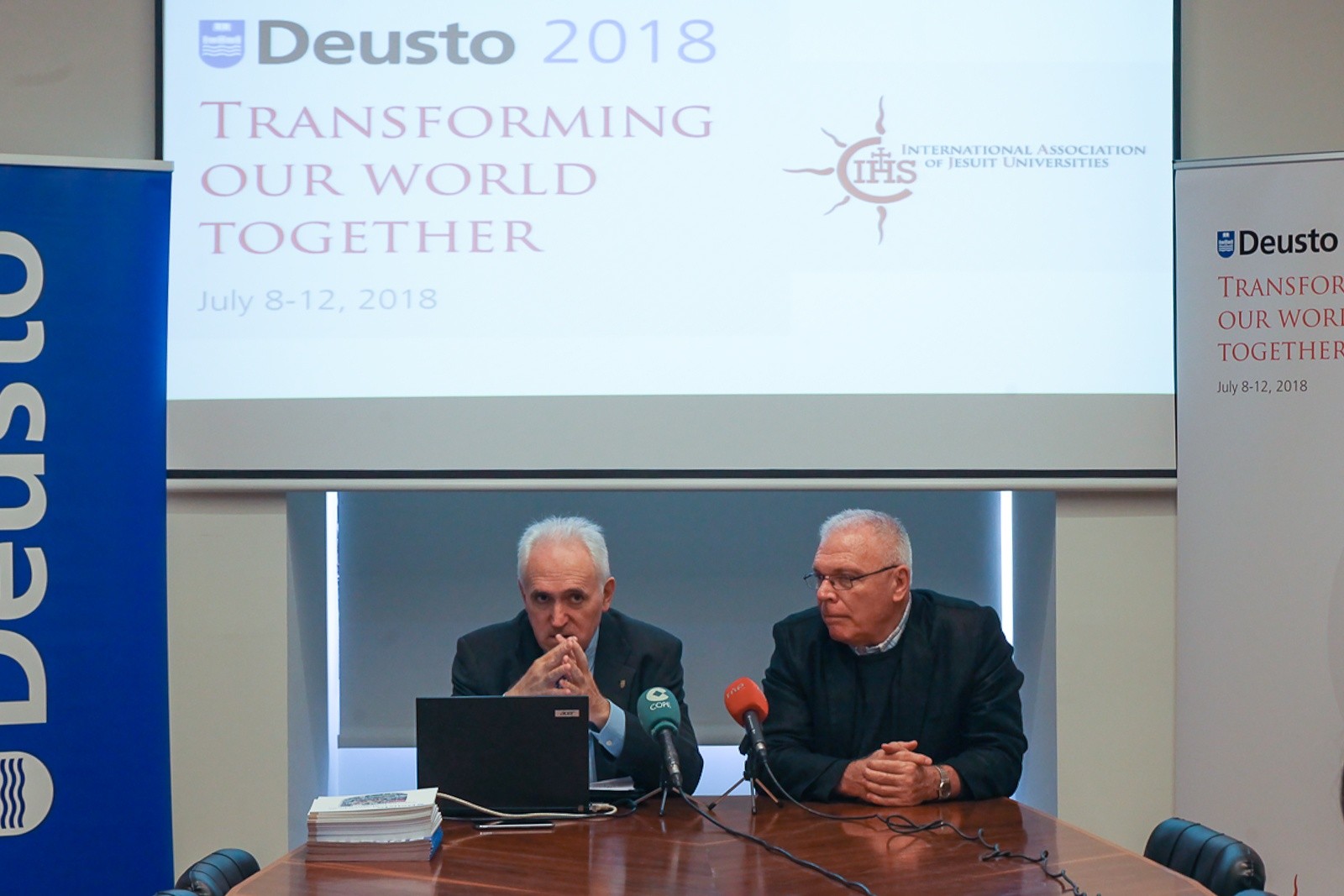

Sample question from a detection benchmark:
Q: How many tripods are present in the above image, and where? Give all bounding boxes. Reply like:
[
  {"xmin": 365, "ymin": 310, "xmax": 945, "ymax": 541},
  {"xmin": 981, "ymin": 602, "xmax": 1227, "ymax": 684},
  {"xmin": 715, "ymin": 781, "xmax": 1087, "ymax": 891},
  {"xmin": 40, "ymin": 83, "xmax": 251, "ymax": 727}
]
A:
[
  {"xmin": 708, "ymin": 734, "xmax": 782, "ymax": 814},
  {"xmin": 634, "ymin": 757, "xmax": 703, "ymax": 815}
]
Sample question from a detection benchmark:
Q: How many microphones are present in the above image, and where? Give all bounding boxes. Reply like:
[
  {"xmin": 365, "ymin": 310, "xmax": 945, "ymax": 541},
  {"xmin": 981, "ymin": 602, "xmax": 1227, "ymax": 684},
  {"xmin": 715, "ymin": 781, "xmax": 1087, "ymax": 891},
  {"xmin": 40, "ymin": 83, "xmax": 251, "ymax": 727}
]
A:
[
  {"xmin": 638, "ymin": 687, "xmax": 684, "ymax": 788},
  {"xmin": 724, "ymin": 677, "xmax": 769, "ymax": 762}
]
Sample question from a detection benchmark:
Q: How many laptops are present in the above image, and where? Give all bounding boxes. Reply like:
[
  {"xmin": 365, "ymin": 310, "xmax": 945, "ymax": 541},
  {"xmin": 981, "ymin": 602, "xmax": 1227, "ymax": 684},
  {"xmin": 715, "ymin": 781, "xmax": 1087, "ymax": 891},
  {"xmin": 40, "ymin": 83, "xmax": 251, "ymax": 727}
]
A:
[{"xmin": 415, "ymin": 695, "xmax": 593, "ymax": 817}]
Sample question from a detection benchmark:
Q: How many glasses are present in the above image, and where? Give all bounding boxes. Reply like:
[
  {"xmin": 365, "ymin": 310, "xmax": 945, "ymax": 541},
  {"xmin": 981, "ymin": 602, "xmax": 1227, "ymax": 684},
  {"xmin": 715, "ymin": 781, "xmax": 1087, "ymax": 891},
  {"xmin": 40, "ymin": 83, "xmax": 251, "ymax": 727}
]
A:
[{"xmin": 803, "ymin": 564, "xmax": 901, "ymax": 590}]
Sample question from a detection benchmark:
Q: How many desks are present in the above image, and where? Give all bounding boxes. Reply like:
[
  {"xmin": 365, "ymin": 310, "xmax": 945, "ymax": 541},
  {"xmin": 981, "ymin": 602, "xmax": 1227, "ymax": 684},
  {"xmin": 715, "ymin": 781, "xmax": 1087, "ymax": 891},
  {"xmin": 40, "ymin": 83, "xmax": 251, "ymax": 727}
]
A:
[{"xmin": 226, "ymin": 797, "xmax": 1225, "ymax": 896}]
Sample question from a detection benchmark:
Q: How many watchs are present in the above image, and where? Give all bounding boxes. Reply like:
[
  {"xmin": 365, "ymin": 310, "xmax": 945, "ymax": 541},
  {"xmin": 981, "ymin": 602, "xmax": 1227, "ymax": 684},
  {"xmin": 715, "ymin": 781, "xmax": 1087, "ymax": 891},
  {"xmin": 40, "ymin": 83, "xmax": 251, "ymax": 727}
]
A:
[{"xmin": 933, "ymin": 764, "xmax": 953, "ymax": 802}]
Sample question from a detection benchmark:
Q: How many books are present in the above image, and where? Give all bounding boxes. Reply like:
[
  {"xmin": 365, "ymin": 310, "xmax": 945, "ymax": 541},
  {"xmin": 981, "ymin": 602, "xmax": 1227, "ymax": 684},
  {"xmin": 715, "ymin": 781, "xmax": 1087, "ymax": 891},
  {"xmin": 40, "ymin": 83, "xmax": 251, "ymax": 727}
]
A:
[{"xmin": 304, "ymin": 787, "xmax": 443, "ymax": 864}]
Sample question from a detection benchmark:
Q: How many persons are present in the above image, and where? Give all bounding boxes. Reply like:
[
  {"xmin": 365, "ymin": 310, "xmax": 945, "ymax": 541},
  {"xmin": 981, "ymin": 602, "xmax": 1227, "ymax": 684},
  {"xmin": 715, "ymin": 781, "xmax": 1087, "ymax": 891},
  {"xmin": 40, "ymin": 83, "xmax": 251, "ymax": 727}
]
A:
[
  {"xmin": 739, "ymin": 509, "xmax": 1028, "ymax": 808},
  {"xmin": 450, "ymin": 516, "xmax": 704, "ymax": 801}
]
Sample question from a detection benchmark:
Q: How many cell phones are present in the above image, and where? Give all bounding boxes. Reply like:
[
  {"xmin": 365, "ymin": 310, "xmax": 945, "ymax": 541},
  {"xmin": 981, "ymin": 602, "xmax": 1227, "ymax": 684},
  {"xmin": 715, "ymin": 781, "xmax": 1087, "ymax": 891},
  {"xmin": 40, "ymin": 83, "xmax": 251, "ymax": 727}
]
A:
[{"xmin": 474, "ymin": 819, "xmax": 554, "ymax": 830}]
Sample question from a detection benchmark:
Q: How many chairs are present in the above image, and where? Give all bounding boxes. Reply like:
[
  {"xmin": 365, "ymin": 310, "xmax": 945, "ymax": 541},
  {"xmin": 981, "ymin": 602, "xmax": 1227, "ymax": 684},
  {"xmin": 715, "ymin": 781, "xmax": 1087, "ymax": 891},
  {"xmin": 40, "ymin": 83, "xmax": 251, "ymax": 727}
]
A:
[
  {"xmin": 153, "ymin": 848, "xmax": 260, "ymax": 896},
  {"xmin": 1143, "ymin": 817, "xmax": 1267, "ymax": 896}
]
